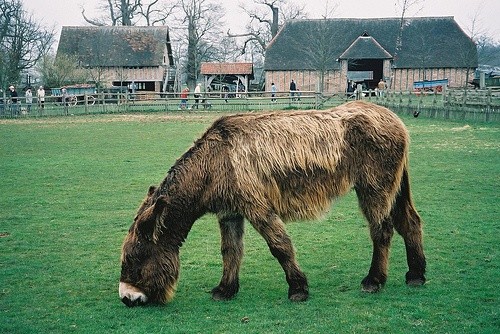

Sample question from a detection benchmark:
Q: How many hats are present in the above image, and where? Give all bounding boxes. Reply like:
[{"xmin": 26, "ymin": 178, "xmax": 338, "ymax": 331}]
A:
[
  {"xmin": 39, "ymin": 86, "xmax": 44, "ymax": 88},
  {"xmin": 9, "ymin": 86, "xmax": 14, "ymax": 88}
]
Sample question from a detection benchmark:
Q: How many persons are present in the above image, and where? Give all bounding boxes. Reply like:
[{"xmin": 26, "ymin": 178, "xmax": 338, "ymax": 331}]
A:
[
  {"xmin": 179, "ymin": 87, "xmax": 191, "ymax": 110},
  {"xmin": 347, "ymin": 81, "xmax": 368, "ymax": 92},
  {"xmin": 296, "ymin": 89, "xmax": 301, "ymax": 101},
  {"xmin": 270, "ymin": 83, "xmax": 277, "ymax": 101},
  {"xmin": 223, "ymin": 85, "xmax": 229, "ymax": 103},
  {"xmin": 26, "ymin": 88, "xmax": 33, "ymax": 111},
  {"xmin": 377, "ymin": 79, "xmax": 388, "ymax": 90},
  {"xmin": 9, "ymin": 86, "xmax": 18, "ymax": 115},
  {"xmin": 37, "ymin": 86, "xmax": 45, "ymax": 110},
  {"xmin": 192, "ymin": 82, "xmax": 201, "ymax": 109},
  {"xmin": 289, "ymin": 79, "xmax": 296, "ymax": 101}
]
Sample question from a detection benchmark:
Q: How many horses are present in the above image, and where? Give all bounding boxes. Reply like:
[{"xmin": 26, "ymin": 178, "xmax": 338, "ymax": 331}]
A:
[{"xmin": 118, "ymin": 98, "xmax": 426, "ymax": 307}]
